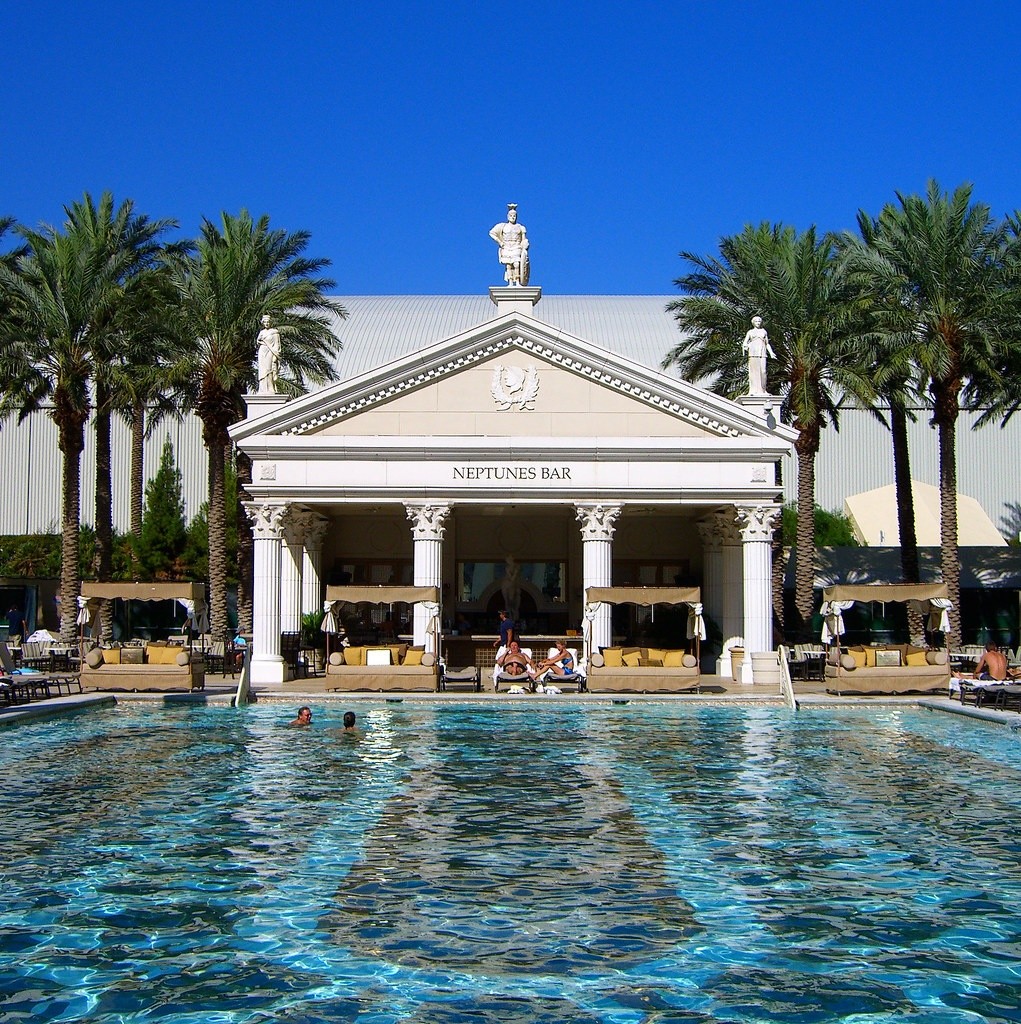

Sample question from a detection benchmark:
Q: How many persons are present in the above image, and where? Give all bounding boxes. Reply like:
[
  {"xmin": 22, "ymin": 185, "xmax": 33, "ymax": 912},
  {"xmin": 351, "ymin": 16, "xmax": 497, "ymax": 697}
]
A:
[
  {"xmin": 743, "ymin": 317, "xmax": 777, "ymax": 396},
  {"xmin": 257, "ymin": 315, "xmax": 282, "ymax": 395},
  {"xmin": 496, "ymin": 640, "xmax": 537, "ymax": 676},
  {"xmin": 488, "ymin": 204, "xmax": 529, "ymax": 286},
  {"xmin": 288, "ymin": 706, "xmax": 312, "ymax": 725},
  {"xmin": 526, "ymin": 640, "xmax": 573, "ymax": 680},
  {"xmin": 493, "ymin": 609, "xmax": 513, "ymax": 648},
  {"xmin": 229, "ymin": 625, "xmax": 248, "ymax": 664},
  {"xmin": 338, "ymin": 712, "xmax": 358, "ymax": 732},
  {"xmin": 952, "ymin": 641, "xmax": 1006, "ymax": 681},
  {"xmin": 501, "ymin": 554, "xmax": 522, "ymax": 622}
]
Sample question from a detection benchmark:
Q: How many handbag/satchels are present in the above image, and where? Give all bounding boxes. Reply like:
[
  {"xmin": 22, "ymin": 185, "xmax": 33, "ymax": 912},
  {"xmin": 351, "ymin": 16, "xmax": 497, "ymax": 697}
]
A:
[{"xmin": 512, "ymin": 631, "xmax": 520, "ymax": 643}]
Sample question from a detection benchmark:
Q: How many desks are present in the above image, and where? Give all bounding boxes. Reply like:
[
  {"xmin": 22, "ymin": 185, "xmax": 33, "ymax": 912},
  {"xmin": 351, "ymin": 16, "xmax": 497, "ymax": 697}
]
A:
[
  {"xmin": 294, "ymin": 647, "xmax": 316, "ymax": 677},
  {"xmin": 802, "ymin": 650, "xmax": 829, "ymax": 682},
  {"xmin": 44, "ymin": 647, "xmax": 75, "ymax": 675},
  {"xmin": 950, "ymin": 652, "xmax": 982, "ymax": 665}
]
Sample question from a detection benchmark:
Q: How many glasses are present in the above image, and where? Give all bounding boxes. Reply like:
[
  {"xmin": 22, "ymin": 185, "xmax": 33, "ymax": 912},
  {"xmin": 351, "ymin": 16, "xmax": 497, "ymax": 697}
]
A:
[{"xmin": 305, "ymin": 713, "xmax": 312, "ymax": 717}]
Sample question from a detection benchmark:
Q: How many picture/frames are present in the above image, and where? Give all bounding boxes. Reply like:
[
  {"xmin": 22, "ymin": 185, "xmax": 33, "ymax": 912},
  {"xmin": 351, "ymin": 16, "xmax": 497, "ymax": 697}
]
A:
[{"xmin": 366, "ymin": 648, "xmax": 391, "ymax": 665}]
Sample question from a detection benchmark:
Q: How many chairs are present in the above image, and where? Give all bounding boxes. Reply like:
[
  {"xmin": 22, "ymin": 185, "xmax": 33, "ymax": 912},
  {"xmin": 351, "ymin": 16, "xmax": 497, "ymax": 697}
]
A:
[
  {"xmin": 206, "ymin": 640, "xmax": 235, "ymax": 679},
  {"xmin": 0, "ymin": 640, "xmax": 94, "ymax": 709},
  {"xmin": 945, "ymin": 679, "xmax": 1021, "ymax": 713},
  {"xmin": 539, "ymin": 648, "xmax": 587, "ymax": 694},
  {"xmin": 784, "ymin": 643, "xmax": 825, "ymax": 682},
  {"xmin": 493, "ymin": 646, "xmax": 533, "ymax": 693},
  {"xmin": 281, "ymin": 630, "xmax": 301, "ymax": 681},
  {"xmin": 436, "ymin": 632, "xmax": 481, "ymax": 692}
]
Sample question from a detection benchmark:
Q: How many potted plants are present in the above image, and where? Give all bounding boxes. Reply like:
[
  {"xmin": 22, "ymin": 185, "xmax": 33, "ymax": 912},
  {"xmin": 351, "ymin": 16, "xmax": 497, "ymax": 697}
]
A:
[{"xmin": 729, "ymin": 645, "xmax": 745, "ymax": 682}]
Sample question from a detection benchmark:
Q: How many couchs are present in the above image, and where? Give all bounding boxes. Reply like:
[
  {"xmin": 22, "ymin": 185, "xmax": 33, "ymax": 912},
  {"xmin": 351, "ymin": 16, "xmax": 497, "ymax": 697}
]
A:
[
  {"xmin": 824, "ymin": 651, "xmax": 951, "ymax": 697},
  {"xmin": 325, "ymin": 652, "xmax": 437, "ymax": 693},
  {"xmin": 585, "ymin": 653, "xmax": 700, "ymax": 695},
  {"xmin": 79, "ymin": 650, "xmax": 207, "ymax": 693}
]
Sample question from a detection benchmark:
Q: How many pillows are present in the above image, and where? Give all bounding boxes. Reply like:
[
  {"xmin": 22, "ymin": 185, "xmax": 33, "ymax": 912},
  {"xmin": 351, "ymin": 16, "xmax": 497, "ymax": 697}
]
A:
[
  {"xmin": 847, "ymin": 645, "xmax": 931, "ymax": 667},
  {"xmin": 598, "ymin": 646, "xmax": 684, "ymax": 667},
  {"xmin": 81, "ymin": 641, "xmax": 190, "ymax": 668},
  {"xmin": 343, "ymin": 643, "xmax": 426, "ymax": 666}
]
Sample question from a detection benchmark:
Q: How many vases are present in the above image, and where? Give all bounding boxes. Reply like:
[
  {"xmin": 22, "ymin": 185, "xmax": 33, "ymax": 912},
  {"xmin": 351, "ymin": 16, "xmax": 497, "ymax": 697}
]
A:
[{"xmin": 751, "ymin": 652, "xmax": 781, "ymax": 685}]
